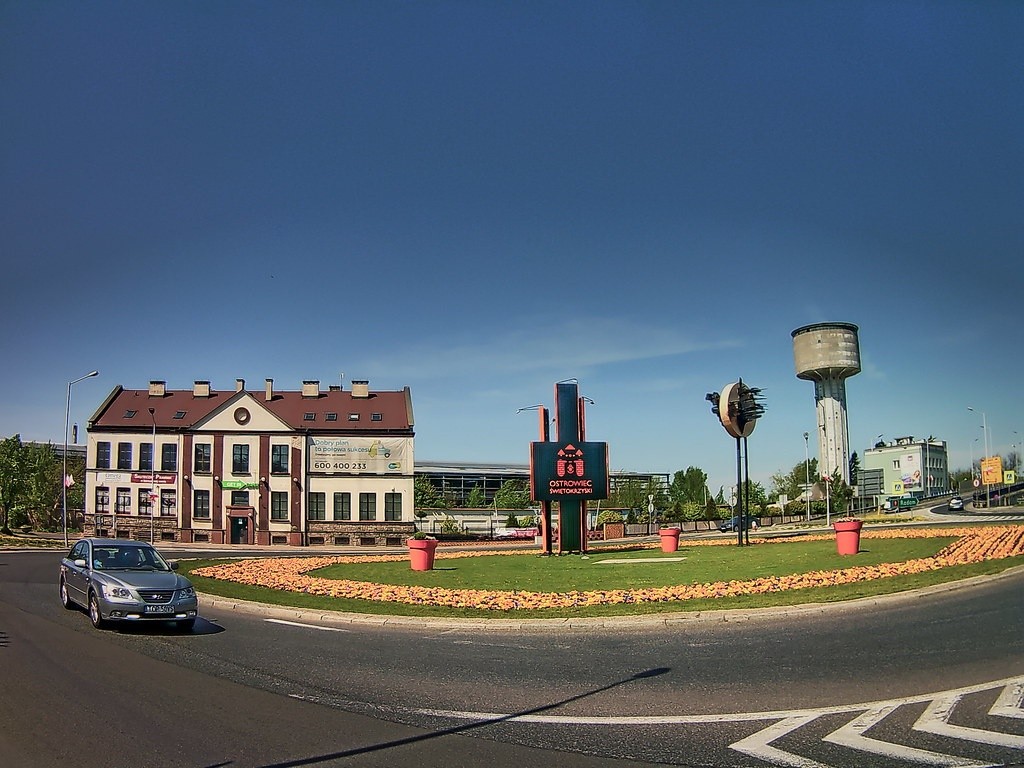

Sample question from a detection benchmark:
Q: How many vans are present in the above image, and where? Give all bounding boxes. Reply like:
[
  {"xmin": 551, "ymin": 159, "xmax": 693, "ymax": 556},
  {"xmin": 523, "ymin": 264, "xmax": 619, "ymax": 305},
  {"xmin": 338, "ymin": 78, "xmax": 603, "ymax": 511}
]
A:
[{"xmin": 884, "ymin": 494, "xmax": 911, "ymax": 514}]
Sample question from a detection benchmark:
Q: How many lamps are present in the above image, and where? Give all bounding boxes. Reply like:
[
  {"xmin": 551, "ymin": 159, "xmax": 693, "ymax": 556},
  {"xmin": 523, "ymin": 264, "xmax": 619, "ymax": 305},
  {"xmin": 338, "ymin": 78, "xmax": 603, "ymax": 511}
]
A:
[
  {"xmin": 292, "ymin": 477, "xmax": 299, "ymax": 482},
  {"xmin": 248, "ymin": 512, "xmax": 252, "ymax": 517},
  {"xmin": 260, "ymin": 476, "xmax": 266, "ymax": 482},
  {"xmin": 184, "ymin": 474, "xmax": 189, "ymax": 480},
  {"xmin": 226, "ymin": 512, "xmax": 230, "ymax": 517},
  {"xmin": 214, "ymin": 475, "xmax": 220, "ymax": 481}
]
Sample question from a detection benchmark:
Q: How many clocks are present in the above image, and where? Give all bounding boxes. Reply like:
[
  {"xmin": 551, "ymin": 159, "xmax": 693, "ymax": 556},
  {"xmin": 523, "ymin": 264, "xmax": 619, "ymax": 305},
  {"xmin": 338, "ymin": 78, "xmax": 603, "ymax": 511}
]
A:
[{"xmin": 234, "ymin": 407, "xmax": 251, "ymax": 425}]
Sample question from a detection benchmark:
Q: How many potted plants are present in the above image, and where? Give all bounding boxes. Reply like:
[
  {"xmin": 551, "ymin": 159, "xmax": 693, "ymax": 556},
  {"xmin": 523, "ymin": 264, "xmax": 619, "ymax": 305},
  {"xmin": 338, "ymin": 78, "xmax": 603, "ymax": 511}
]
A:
[
  {"xmin": 659, "ymin": 523, "xmax": 680, "ymax": 552},
  {"xmin": 406, "ymin": 532, "xmax": 438, "ymax": 571}
]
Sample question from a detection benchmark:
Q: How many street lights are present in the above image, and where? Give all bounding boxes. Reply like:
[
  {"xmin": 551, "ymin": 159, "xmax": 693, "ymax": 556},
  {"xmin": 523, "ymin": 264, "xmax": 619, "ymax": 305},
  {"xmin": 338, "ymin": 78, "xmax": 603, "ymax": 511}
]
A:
[
  {"xmin": 63, "ymin": 370, "xmax": 99, "ymax": 548},
  {"xmin": 148, "ymin": 407, "xmax": 157, "ymax": 544},
  {"xmin": 803, "ymin": 425, "xmax": 825, "ymax": 521},
  {"xmin": 967, "ymin": 407, "xmax": 993, "ymax": 509},
  {"xmin": 516, "ymin": 404, "xmax": 542, "ymax": 414},
  {"xmin": 927, "ymin": 435, "xmax": 932, "ymax": 500},
  {"xmin": 872, "ymin": 434, "xmax": 884, "ymax": 511},
  {"xmin": 582, "ymin": 395, "xmax": 595, "ymax": 405}
]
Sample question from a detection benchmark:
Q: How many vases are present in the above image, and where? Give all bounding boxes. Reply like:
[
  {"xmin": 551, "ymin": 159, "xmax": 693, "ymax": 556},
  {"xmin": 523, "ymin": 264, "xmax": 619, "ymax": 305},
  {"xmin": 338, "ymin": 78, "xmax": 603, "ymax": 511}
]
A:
[{"xmin": 832, "ymin": 520, "xmax": 863, "ymax": 555}]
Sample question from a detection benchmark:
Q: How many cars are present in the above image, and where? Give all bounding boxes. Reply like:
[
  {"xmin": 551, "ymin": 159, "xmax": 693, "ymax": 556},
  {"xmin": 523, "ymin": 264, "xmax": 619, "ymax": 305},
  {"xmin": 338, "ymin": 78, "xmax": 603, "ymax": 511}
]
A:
[
  {"xmin": 946, "ymin": 496, "xmax": 964, "ymax": 510},
  {"xmin": 59, "ymin": 540, "xmax": 198, "ymax": 629},
  {"xmin": 719, "ymin": 515, "xmax": 760, "ymax": 533}
]
